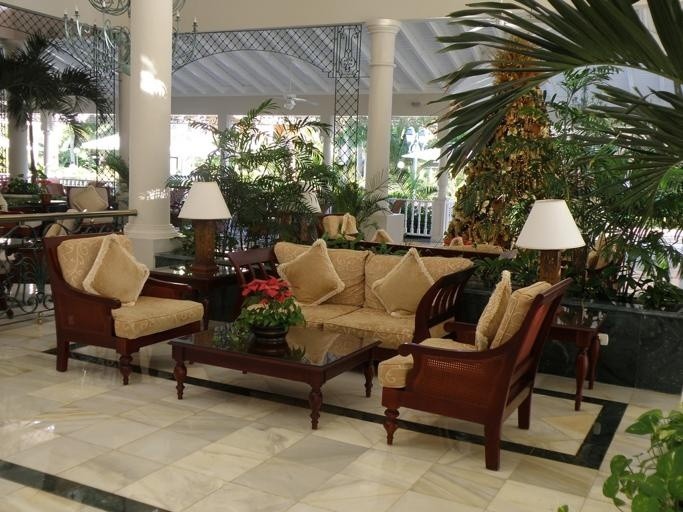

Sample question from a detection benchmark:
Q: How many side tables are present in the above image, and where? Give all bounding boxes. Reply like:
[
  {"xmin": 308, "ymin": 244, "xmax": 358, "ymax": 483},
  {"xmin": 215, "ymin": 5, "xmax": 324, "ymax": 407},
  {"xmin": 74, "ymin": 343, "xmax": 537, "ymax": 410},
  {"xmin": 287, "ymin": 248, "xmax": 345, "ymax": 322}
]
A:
[
  {"xmin": 151, "ymin": 263, "xmax": 250, "ymax": 318},
  {"xmin": 552, "ymin": 305, "xmax": 608, "ymax": 413}
]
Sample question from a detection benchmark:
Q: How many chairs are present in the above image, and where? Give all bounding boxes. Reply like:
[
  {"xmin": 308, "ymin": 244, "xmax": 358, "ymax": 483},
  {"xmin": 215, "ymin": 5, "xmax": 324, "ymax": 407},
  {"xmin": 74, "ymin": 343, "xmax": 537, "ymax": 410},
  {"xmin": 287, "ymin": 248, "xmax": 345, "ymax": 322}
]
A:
[
  {"xmin": 381, "ymin": 276, "xmax": 574, "ymax": 472},
  {"xmin": 67, "ymin": 185, "xmax": 117, "ymax": 232},
  {"xmin": 42, "ymin": 231, "xmax": 205, "ymax": 388},
  {"xmin": 314, "ymin": 213, "xmax": 358, "ymax": 238}
]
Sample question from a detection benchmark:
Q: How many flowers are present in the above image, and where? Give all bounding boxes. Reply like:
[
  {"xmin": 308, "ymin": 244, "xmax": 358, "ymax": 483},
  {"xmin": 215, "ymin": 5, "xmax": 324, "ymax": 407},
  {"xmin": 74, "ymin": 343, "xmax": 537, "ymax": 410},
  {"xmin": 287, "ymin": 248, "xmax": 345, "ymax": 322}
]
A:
[{"xmin": 235, "ymin": 277, "xmax": 308, "ymax": 333}]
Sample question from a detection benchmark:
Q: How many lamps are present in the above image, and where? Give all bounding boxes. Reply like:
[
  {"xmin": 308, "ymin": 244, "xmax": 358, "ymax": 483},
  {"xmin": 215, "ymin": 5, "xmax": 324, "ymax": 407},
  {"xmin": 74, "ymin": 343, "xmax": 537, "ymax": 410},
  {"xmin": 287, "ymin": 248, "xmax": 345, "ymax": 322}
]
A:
[
  {"xmin": 178, "ymin": 181, "xmax": 232, "ymax": 274},
  {"xmin": 512, "ymin": 198, "xmax": 588, "ymax": 285}
]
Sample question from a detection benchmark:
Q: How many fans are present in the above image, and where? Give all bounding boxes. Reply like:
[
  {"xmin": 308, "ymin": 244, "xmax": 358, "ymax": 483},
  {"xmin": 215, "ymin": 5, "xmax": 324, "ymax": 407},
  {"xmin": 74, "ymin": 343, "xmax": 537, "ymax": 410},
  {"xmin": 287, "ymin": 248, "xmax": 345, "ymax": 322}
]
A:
[{"xmin": 267, "ymin": 56, "xmax": 320, "ymax": 111}]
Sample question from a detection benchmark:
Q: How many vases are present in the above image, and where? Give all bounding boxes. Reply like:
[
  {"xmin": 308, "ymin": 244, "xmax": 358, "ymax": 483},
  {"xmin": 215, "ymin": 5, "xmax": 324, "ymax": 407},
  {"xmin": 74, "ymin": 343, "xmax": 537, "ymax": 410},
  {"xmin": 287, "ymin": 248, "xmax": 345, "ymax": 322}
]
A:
[
  {"xmin": 249, "ymin": 322, "xmax": 289, "ymax": 342},
  {"xmin": 41, "ymin": 193, "xmax": 52, "ymax": 205}
]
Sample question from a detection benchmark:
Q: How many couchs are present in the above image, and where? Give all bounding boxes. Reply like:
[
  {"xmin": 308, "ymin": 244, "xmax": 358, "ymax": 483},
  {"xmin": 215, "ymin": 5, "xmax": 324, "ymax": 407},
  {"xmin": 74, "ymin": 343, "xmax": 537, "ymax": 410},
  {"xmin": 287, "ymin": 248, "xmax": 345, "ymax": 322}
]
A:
[{"xmin": 228, "ymin": 245, "xmax": 481, "ymax": 363}]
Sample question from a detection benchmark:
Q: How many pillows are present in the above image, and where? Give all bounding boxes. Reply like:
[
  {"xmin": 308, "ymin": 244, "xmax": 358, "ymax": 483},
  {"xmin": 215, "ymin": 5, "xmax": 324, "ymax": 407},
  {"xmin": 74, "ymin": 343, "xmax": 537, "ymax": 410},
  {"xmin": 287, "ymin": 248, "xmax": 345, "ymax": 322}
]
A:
[
  {"xmin": 475, "ymin": 270, "xmax": 512, "ymax": 351},
  {"xmin": 82, "ymin": 234, "xmax": 150, "ymax": 308},
  {"xmin": 73, "ymin": 183, "xmax": 108, "ymax": 212},
  {"xmin": 340, "ymin": 211, "xmax": 358, "ymax": 235},
  {"xmin": 275, "ymin": 239, "xmax": 436, "ymax": 320}
]
